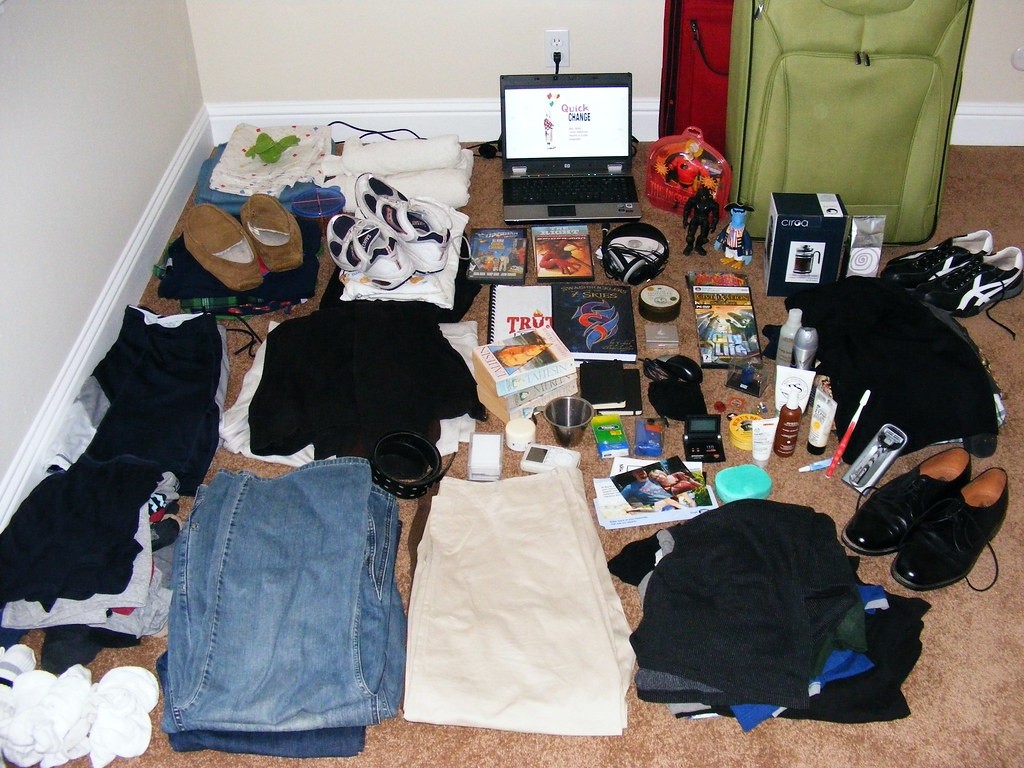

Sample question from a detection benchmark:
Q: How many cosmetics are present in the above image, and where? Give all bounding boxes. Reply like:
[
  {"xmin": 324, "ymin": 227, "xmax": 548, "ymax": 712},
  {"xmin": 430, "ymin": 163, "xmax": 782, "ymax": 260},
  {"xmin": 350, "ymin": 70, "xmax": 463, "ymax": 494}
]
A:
[
  {"xmin": 751, "ymin": 416, "xmax": 780, "ymax": 468},
  {"xmin": 773, "ymin": 308, "xmax": 838, "ymax": 458}
]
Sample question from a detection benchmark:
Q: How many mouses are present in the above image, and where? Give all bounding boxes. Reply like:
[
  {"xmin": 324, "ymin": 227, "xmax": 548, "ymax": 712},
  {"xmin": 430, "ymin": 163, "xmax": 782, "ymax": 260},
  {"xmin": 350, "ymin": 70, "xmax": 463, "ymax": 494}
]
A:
[{"xmin": 655, "ymin": 354, "xmax": 704, "ymax": 384}]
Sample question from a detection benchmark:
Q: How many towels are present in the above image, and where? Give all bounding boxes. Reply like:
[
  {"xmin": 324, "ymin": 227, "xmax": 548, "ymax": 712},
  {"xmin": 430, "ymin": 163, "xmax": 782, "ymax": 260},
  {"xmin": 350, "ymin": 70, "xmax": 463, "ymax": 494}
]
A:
[{"xmin": 320, "ymin": 134, "xmax": 474, "ymax": 213}]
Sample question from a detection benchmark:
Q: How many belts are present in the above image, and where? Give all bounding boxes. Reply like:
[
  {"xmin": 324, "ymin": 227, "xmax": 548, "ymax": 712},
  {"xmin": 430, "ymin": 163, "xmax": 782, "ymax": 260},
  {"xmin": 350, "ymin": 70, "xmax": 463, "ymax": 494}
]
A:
[{"xmin": 372, "ymin": 431, "xmax": 457, "ymax": 499}]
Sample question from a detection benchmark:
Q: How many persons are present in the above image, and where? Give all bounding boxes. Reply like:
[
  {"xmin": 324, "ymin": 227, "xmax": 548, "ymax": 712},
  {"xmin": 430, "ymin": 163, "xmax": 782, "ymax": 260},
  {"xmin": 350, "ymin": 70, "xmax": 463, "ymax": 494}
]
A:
[
  {"xmin": 714, "ymin": 203, "xmax": 753, "ymax": 270},
  {"xmin": 682, "ymin": 185, "xmax": 720, "ymax": 256},
  {"xmin": 616, "ymin": 466, "xmax": 714, "ymax": 513}
]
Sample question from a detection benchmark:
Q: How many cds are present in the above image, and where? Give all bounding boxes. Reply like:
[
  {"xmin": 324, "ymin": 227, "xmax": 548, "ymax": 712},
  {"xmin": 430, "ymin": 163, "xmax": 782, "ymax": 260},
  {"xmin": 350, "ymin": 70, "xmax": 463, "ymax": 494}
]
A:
[{"xmin": 641, "ymin": 285, "xmax": 679, "ymax": 307}]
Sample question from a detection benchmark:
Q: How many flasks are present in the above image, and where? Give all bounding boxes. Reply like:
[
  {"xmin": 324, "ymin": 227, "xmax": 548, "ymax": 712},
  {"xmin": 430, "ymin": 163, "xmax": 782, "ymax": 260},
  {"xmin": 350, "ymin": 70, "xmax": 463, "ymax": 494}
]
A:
[{"xmin": 791, "ymin": 327, "xmax": 818, "ymax": 371}]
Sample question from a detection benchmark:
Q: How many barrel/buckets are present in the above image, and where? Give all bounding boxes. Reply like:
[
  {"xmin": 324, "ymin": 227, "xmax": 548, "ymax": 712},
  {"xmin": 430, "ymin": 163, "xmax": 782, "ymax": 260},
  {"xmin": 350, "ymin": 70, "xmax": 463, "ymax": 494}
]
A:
[{"xmin": 543, "ymin": 396, "xmax": 594, "ymax": 448}]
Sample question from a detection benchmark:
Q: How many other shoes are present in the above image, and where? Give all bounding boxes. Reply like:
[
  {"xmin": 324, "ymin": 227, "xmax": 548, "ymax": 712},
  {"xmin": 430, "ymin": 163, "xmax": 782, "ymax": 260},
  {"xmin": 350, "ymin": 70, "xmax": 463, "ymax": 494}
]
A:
[
  {"xmin": 241, "ymin": 193, "xmax": 304, "ymax": 271},
  {"xmin": 186, "ymin": 204, "xmax": 263, "ymax": 291}
]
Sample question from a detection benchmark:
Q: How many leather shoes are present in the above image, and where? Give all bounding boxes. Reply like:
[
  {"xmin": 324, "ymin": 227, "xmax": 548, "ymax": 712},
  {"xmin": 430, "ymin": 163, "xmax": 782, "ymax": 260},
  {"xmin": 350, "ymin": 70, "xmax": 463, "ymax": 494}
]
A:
[
  {"xmin": 841, "ymin": 447, "xmax": 972, "ymax": 556},
  {"xmin": 890, "ymin": 467, "xmax": 1010, "ymax": 590}
]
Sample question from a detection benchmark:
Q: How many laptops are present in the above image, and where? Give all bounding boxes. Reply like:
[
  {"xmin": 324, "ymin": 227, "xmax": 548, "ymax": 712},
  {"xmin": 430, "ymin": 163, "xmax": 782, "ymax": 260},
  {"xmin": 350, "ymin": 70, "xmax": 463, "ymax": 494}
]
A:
[{"xmin": 500, "ymin": 73, "xmax": 641, "ymax": 226}]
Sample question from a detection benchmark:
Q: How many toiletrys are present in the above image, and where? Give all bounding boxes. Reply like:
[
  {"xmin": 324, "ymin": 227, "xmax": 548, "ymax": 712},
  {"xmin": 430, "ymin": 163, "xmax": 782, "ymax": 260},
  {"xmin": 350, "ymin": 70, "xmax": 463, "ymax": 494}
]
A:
[
  {"xmin": 638, "ymin": 283, "xmax": 682, "ymax": 324},
  {"xmin": 772, "ymin": 308, "xmax": 818, "ymax": 390},
  {"xmin": 728, "ymin": 364, "xmax": 838, "ymax": 470}
]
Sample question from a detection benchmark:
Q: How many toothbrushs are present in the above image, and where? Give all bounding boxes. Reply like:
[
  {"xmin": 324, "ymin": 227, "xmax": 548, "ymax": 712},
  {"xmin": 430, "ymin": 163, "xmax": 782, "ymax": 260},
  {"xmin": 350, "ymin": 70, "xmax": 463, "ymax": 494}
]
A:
[{"xmin": 825, "ymin": 389, "xmax": 872, "ymax": 479}]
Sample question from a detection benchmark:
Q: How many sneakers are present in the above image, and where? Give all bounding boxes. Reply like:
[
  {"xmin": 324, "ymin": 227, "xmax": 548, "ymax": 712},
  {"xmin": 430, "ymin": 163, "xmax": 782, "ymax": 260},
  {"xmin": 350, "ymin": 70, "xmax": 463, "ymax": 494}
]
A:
[
  {"xmin": 354, "ymin": 172, "xmax": 451, "ymax": 272},
  {"xmin": 916, "ymin": 246, "xmax": 1024, "ymax": 317},
  {"xmin": 327, "ymin": 214, "xmax": 414, "ymax": 290},
  {"xmin": 884, "ymin": 230, "xmax": 993, "ymax": 295}
]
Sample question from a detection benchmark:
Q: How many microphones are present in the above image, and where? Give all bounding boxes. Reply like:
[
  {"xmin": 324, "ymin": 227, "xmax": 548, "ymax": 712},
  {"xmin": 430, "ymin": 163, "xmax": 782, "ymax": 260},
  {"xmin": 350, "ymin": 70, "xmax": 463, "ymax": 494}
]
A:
[{"xmin": 602, "ymin": 223, "xmax": 610, "ymax": 240}]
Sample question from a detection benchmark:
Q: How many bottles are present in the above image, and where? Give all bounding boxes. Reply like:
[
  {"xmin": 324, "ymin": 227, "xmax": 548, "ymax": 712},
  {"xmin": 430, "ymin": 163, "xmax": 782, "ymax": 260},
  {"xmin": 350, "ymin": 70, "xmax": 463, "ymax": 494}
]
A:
[
  {"xmin": 776, "ymin": 308, "xmax": 803, "ymax": 367},
  {"xmin": 773, "ymin": 390, "xmax": 802, "ymax": 458}
]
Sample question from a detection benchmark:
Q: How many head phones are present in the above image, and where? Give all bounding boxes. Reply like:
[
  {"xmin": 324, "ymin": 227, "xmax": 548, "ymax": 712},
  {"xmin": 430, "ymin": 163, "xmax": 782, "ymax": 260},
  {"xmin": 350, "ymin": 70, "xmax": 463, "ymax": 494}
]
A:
[{"xmin": 602, "ymin": 222, "xmax": 670, "ymax": 285}]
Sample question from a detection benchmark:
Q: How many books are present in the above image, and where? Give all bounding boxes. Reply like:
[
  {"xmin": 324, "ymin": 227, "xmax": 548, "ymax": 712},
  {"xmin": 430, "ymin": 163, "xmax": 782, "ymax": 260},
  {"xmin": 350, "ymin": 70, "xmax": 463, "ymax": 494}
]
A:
[
  {"xmin": 589, "ymin": 414, "xmax": 630, "ymax": 459},
  {"xmin": 466, "ymin": 223, "xmax": 596, "ymax": 286},
  {"xmin": 471, "ymin": 281, "xmax": 644, "ymax": 426},
  {"xmin": 685, "ymin": 269, "xmax": 765, "ymax": 370}
]
